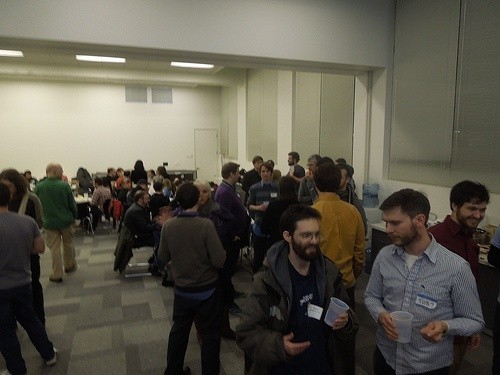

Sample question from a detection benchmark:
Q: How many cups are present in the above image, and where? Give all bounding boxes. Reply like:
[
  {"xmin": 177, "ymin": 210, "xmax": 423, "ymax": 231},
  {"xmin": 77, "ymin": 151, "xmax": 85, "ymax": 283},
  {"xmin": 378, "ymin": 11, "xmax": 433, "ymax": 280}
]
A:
[
  {"xmin": 390, "ymin": 311, "xmax": 413, "ymax": 343},
  {"xmin": 264, "ymin": 202, "xmax": 269, "ymax": 205},
  {"xmin": 324, "ymin": 297, "xmax": 349, "ymax": 326},
  {"xmin": 289, "ymin": 166, "xmax": 294, "ymax": 171}
]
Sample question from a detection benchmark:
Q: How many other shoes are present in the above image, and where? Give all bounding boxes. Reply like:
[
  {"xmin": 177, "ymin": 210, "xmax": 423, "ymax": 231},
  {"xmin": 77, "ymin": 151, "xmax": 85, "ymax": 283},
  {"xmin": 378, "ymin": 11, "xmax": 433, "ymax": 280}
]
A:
[
  {"xmin": 50, "ymin": 275, "xmax": 62, "ymax": 282},
  {"xmin": 221, "ymin": 329, "xmax": 236, "ymax": 340},
  {"xmin": 148, "ymin": 265, "xmax": 163, "ymax": 276},
  {"xmin": 164, "ymin": 367, "xmax": 190, "ymax": 375},
  {"xmin": 45, "ymin": 341, "xmax": 57, "ymax": 365},
  {"xmin": 1, "ymin": 369, "xmax": 12, "ymax": 375}
]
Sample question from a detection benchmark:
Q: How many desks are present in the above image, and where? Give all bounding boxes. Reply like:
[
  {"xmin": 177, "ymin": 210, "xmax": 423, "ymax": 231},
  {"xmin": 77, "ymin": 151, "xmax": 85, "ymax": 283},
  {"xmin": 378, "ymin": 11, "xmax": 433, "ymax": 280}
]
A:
[{"xmin": 371, "ymin": 230, "xmax": 500, "ymax": 338}]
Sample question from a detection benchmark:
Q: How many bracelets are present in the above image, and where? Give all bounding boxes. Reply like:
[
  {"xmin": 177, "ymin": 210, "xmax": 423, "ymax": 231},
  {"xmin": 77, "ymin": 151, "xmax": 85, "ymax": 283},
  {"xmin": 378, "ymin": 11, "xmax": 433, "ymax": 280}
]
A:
[{"xmin": 440, "ymin": 321, "xmax": 447, "ymax": 334}]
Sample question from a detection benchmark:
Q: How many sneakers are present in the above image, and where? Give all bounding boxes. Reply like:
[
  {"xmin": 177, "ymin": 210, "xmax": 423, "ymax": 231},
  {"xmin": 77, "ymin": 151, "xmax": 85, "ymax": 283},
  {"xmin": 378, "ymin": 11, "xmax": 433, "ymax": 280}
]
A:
[
  {"xmin": 229, "ymin": 303, "xmax": 242, "ymax": 315},
  {"xmin": 237, "ymin": 292, "xmax": 246, "ymax": 298}
]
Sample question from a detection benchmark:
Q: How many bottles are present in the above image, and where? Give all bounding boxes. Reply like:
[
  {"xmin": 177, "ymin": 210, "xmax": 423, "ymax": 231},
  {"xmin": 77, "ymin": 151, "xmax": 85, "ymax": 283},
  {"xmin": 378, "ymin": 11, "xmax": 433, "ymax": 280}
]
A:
[{"xmin": 362, "ymin": 183, "xmax": 380, "ymax": 207}]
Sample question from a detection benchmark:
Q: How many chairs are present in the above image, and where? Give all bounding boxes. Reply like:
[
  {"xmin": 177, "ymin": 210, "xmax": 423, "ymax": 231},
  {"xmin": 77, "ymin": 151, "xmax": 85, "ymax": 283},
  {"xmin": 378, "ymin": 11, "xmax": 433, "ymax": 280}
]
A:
[{"xmin": 74, "ymin": 199, "xmax": 160, "ymax": 278}]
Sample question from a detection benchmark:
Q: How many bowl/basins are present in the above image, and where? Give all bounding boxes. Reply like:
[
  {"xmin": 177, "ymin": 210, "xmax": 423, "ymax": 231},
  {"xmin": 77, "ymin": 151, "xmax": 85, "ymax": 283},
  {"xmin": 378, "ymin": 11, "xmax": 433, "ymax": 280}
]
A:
[{"xmin": 473, "ymin": 228, "xmax": 486, "ymax": 243}]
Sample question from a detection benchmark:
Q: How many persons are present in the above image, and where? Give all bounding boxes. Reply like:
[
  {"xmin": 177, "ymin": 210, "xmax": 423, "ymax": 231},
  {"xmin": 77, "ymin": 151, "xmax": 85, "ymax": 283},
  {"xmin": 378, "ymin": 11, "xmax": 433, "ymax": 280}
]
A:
[
  {"xmin": 363, "ymin": 189, "xmax": 485, "ymax": 375},
  {"xmin": 0, "ymin": 152, "xmax": 500, "ymax": 375},
  {"xmin": 237, "ymin": 204, "xmax": 359, "ymax": 375}
]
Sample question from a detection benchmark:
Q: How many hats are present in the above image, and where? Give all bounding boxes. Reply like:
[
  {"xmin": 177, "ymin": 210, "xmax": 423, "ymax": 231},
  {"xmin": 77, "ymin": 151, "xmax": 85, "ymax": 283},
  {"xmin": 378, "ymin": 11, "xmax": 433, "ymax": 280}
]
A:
[{"xmin": 138, "ymin": 179, "xmax": 150, "ymax": 184}]
